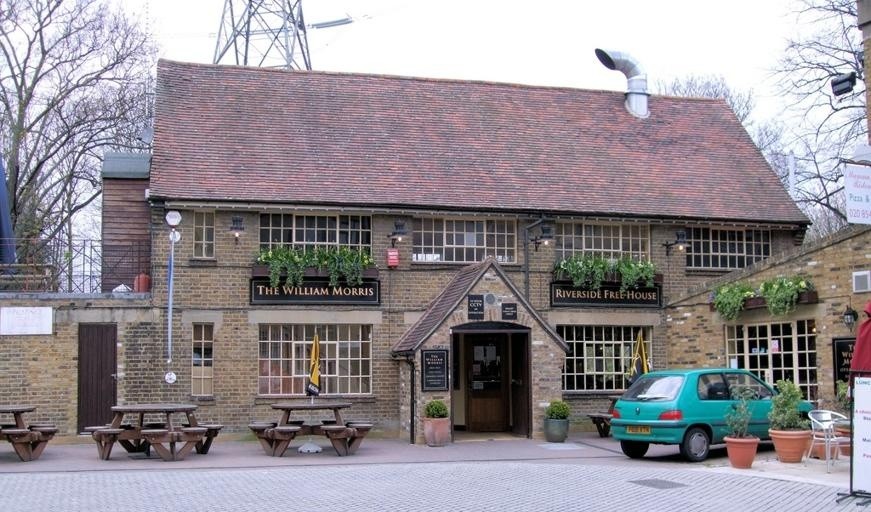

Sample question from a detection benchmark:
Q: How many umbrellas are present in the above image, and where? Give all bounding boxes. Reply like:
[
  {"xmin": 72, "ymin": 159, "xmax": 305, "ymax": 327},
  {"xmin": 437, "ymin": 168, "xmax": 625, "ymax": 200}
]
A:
[
  {"xmin": 304, "ymin": 325, "xmax": 322, "ymax": 443},
  {"xmin": 0, "ymin": 152, "xmax": 20, "ymax": 277},
  {"xmin": 845, "ymin": 301, "xmax": 871, "ymax": 400}
]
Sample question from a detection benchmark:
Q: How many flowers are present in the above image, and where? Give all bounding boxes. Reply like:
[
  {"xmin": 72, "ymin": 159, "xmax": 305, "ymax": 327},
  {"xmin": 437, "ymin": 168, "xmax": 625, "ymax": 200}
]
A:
[
  {"xmin": 706, "ymin": 276, "xmax": 818, "ymax": 321},
  {"xmin": 252, "ymin": 244, "xmax": 379, "ymax": 289},
  {"xmin": 551, "ymin": 254, "xmax": 664, "ymax": 293}
]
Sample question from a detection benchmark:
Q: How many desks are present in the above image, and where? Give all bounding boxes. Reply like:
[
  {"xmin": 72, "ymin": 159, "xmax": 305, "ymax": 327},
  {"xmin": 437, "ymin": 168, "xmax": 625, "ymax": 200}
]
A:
[
  {"xmin": 0, "ymin": 406, "xmax": 35, "ymax": 461},
  {"xmin": 271, "ymin": 403, "xmax": 351, "ymax": 456},
  {"xmin": 104, "ymin": 404, "xmax": 204, "ymax": 462},
  {"xmin": 608, "ymin": 395, "xmax": 622, "ymax": 413}
]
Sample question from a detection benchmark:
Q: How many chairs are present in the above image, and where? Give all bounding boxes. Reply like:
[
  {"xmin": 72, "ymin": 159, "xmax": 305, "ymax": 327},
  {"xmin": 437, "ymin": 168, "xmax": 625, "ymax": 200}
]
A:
[
  {"xmin": 709, "ymin": 382, "xmax": 728, "ymax": 399},
  {"xmin": 808, "ymin": 410, "xmax": 851, "ymax": 473}
]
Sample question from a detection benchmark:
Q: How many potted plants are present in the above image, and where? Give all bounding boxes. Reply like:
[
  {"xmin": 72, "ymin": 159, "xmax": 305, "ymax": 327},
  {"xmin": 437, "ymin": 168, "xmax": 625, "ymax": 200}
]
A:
[
  {"xmin": 423, "ymin": 400, "xmax": 449, "ymax": 447},
  {"xmin": 722, "ymin": 380, "xmax": 850, "ymax": 468},
  {"xmin": 543, "ymin": 401, "xmax": 569, "ymax": 442}
]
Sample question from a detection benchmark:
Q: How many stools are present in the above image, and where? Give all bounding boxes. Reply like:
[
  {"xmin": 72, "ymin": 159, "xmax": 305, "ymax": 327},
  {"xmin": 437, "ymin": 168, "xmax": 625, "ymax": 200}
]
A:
[
  {"xmin": 587, "ymin": 413, "xmax": 612, "ymax": 437},
  {"xmin": 2, "ymin": 421, "xmax": 58, "ymax": 461},
  {"xmin": 247, "ymin": 421, "xmax": 374, "ymax": 457},
  {"xmin": 84, "ymin": 424, "xmax": 225, "ymax": 461}
]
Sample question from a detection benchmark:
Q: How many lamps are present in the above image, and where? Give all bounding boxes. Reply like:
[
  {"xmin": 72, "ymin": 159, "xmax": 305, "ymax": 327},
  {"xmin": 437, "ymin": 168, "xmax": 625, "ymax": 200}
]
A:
[
  {"xmin": 230, "ymin": 216, "xmax": 245, "ymax": 244},
  {"xmin": 387, "ymin": 219, "xmax": 407, "ymax": 248},
  {"xmin": 843, "ymin": 304, "xmax": 858, "ymax": 333},
  {"xmin": 663, "ymin": 231, "xmax": 691, "ymax": 256},
  {"xmin": 530, "ymin": 224, "xmax": 554, "ymax": 252}
]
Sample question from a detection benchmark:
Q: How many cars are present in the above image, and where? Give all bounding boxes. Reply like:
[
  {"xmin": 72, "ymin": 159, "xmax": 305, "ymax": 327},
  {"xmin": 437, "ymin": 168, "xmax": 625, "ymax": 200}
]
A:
[{"xmin": 609, "ymin": 368, "xmax": 815, "ymax": 462}]
